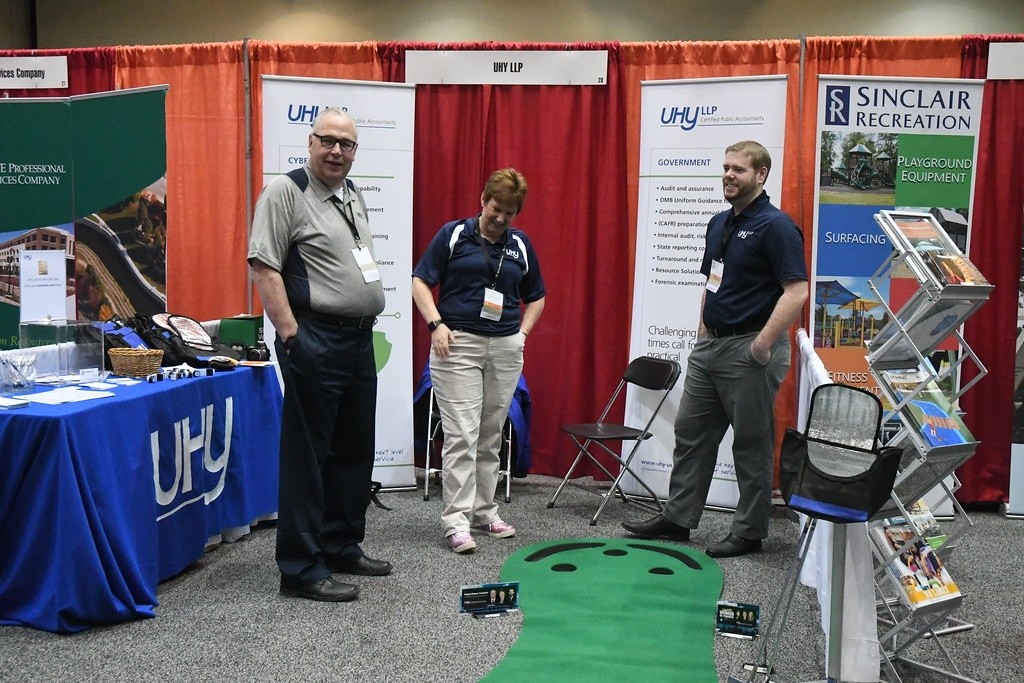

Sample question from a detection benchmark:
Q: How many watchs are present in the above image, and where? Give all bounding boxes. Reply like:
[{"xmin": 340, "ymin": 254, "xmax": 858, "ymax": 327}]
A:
[{"xmin": 427, "ymin": 320, "xmax": 442, "ymax": 332}]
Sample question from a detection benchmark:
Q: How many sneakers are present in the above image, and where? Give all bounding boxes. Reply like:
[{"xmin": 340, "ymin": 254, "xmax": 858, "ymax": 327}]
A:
[
  {"xmin": 470, "ymin": 519, "xmax": 515, "ymax": 537},
  {"xmin": 447, "ymin": 530, "xmax": 476, "ymax": 552}
]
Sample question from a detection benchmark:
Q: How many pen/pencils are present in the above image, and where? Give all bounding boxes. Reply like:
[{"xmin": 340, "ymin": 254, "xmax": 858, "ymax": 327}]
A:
[{"xmin": 0, "ymin": 352, "xmax": 37, "ymax": 390}]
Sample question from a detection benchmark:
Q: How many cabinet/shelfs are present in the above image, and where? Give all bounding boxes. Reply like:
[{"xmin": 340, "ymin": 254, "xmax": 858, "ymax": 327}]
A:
[{"xmin": 863, "ymin": 209, "xmax": 996, "ymax": 683}]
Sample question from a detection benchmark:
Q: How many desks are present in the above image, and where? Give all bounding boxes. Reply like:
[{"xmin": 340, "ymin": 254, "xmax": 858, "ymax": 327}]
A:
[{"xmin": 0, "ymin": 357, "xmax": 284, "ymax": 635}]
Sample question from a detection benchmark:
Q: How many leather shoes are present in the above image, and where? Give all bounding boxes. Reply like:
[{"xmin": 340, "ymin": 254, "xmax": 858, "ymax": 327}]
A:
[
  {"xmin": 706, "ymin": 532, "xmax": 761, "ymax": 557},
  {"xmin": 328, "ymin": 553, "xmax": 393, "ymax": 574},
  {"xmin": 622, "ymin": 514, "xmax": 689, "ymax": 541},
  {"xmin": 279, "ymin": 575, "xmax": 359, "ymax": 600}
]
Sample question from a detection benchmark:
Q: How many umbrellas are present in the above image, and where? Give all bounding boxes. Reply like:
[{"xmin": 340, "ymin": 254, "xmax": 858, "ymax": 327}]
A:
[{"xmin": 816, "ymin": 280, "xmax": 881, "ymax": 348}]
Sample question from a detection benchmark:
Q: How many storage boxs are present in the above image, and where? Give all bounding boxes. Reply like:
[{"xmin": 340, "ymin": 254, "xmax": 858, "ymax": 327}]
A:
[{"xmin": 219, "ymin": 316, "xmax": 264, "ymax": 349}]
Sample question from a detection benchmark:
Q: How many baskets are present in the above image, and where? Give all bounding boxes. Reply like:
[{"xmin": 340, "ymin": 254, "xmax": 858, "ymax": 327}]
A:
[{"xmin": 108, "ymin": 347, "xmax": 164, "ymax": 375}]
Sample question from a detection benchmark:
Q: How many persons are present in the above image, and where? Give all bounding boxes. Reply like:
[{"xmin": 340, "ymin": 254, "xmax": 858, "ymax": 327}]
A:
[
  {"xmin": 622, "ymin": 141, "xmax": 809, "ymax": 558},
  {"xmin": 907, "ymin": 540, "xmax": 937, "ymax": 578},
  {"xmin": 245, "ymin": 108, "xmax": 394, "ymax": 601},
  {"xmin": 884, "ymin": 526, "xmax": 914, "ymax": 567},
  {"xmin": 412, "ymin": 168, "xmax": 547, "ymax": 553}
]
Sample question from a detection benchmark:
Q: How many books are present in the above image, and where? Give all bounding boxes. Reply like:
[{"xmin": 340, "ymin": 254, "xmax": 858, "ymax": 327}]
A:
[
  {"xmin": 869, "ymin": 497, "xmax": 958, "ymax": 610},
  {"xmin": 895, "ymin": 220, "xmax": 989, "ymax": 287},
  {"xmin": 883, "ymin": 370, "xmax": 976, "ymax": 449}
]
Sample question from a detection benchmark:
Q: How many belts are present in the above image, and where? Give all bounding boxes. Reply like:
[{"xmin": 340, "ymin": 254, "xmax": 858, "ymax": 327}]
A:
[
  {"xmin": 707, "ymin": 322, "xmax": 765, "ymax": 336},
  {"xmin": 297, "ymin": 311, "xmax": 377, "ymax": 329}
]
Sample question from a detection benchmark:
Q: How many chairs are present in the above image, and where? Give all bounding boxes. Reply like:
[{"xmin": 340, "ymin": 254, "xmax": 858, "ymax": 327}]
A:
[
  {"xmin": 547, "ymin": 356, "xmax": 682, "ymax": 527},
  {"xmin": 412, "ymin": 355, "xmax": 531, "ymax": 504}
]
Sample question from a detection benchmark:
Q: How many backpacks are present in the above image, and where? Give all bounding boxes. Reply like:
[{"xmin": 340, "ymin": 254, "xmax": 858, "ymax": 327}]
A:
[{"xmin": 128, "ymin": 311, "xmax": 241, "ymax": 366}]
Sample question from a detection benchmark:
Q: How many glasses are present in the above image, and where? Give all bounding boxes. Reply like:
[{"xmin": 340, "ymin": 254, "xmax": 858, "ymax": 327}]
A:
[{"xmin": 313, "ymin": 132, "xmax": 357, "ymax": 151}]
